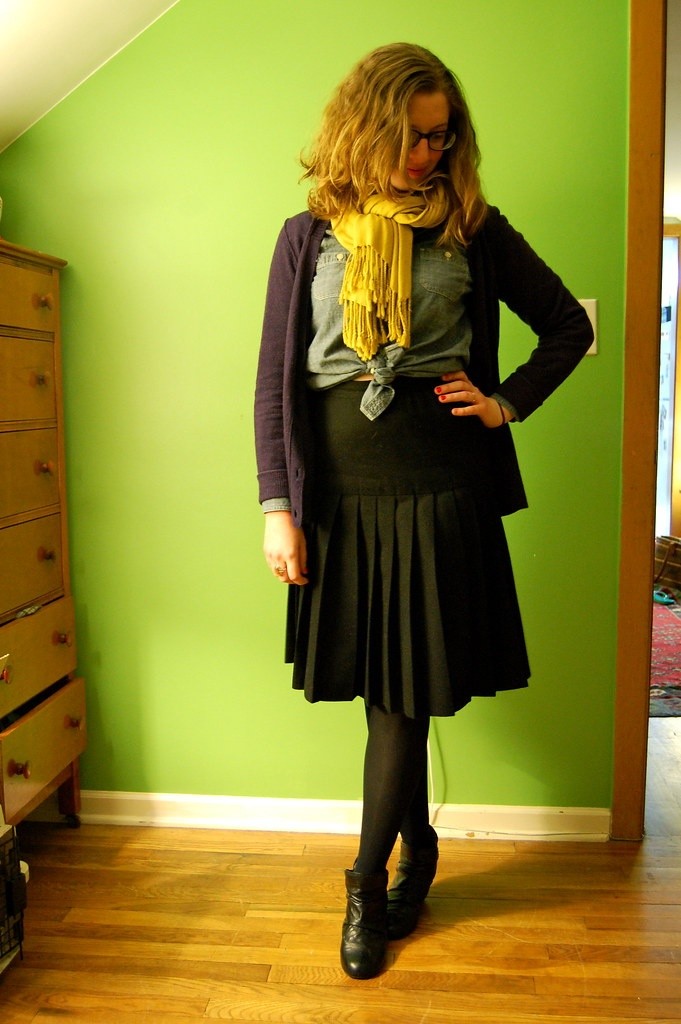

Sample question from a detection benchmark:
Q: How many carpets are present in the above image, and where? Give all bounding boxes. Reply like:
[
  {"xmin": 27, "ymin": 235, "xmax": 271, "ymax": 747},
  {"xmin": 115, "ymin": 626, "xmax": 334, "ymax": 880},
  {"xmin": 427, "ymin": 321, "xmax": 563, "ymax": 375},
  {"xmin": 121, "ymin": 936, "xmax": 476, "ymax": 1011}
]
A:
[{"xmin": 649, "ymin": 602, "xmax": 681, "ymax": 718}]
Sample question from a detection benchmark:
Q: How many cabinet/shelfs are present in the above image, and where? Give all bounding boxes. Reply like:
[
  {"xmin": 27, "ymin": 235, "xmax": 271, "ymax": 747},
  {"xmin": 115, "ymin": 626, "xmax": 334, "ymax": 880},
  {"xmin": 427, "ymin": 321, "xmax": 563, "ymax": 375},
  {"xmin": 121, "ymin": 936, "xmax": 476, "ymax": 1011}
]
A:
[{"xmin": 0, "ymin": 238, "xmax": 88, "ymax": 827}]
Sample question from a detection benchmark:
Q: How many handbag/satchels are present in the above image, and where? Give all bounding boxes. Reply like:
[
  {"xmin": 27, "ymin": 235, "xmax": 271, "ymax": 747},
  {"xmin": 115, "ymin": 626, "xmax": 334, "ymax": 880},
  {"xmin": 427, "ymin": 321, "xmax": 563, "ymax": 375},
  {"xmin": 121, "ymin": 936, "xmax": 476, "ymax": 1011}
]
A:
[{"xmin": 654, "ymin": 535, "xmax": 681, "ymax": 591}]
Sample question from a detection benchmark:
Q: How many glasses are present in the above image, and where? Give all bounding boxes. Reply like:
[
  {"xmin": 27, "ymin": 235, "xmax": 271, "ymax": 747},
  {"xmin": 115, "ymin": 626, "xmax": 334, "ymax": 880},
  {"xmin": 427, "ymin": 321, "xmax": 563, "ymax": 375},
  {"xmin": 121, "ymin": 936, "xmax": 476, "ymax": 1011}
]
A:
[{"xmin": 408, "ymin": 129, "xmax": 457, "ymax": 151}]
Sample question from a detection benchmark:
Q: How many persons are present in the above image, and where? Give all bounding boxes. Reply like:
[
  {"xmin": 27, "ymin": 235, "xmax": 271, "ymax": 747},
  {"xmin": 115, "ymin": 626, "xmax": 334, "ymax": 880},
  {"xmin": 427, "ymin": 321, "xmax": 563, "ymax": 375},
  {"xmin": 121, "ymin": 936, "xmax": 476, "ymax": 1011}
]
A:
[{"xmin": 252, "ymin": 44, "xmax": 593, "ymax": 981}]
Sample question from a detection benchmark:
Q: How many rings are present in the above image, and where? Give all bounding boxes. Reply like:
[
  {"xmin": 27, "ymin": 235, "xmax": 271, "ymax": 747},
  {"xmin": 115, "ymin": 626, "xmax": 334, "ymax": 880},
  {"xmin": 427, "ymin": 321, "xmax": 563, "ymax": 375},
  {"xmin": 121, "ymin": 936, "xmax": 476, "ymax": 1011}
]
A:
[
  {"xmin": 471, "ymin": 392, "xmax": 478, "ymax": 403},
  {"xmin": 275, "ymin": 565, "xmax": 286, "ymax": 576}
]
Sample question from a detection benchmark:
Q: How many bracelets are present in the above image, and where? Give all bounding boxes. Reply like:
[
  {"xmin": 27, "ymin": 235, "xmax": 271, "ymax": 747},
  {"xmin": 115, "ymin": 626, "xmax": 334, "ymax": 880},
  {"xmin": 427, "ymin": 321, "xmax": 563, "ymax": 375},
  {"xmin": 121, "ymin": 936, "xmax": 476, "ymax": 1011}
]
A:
[{"xmin": 495, "ymin": 394, "xmax": 507, "ymax": 430}]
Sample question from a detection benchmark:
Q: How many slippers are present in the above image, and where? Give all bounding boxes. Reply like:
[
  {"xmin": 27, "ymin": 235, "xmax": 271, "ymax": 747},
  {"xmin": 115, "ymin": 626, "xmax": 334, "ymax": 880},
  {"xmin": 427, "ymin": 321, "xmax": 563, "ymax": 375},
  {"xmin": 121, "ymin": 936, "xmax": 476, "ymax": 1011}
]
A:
[{"xmin": 653, "ymin": 591, "xmax": 674, "ymax": 605}]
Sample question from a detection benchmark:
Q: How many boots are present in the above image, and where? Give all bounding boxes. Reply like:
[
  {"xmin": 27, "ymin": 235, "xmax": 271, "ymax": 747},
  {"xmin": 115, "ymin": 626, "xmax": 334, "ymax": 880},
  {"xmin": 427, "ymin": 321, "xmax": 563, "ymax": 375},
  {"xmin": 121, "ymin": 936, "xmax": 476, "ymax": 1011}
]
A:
[
  {"xmin": 339, "ymin": 856, "xmax": 389, "ymax": 980},
  {"xmin": 386, "ymin": 824, "xmax": 438, "ymax": 942}
]
